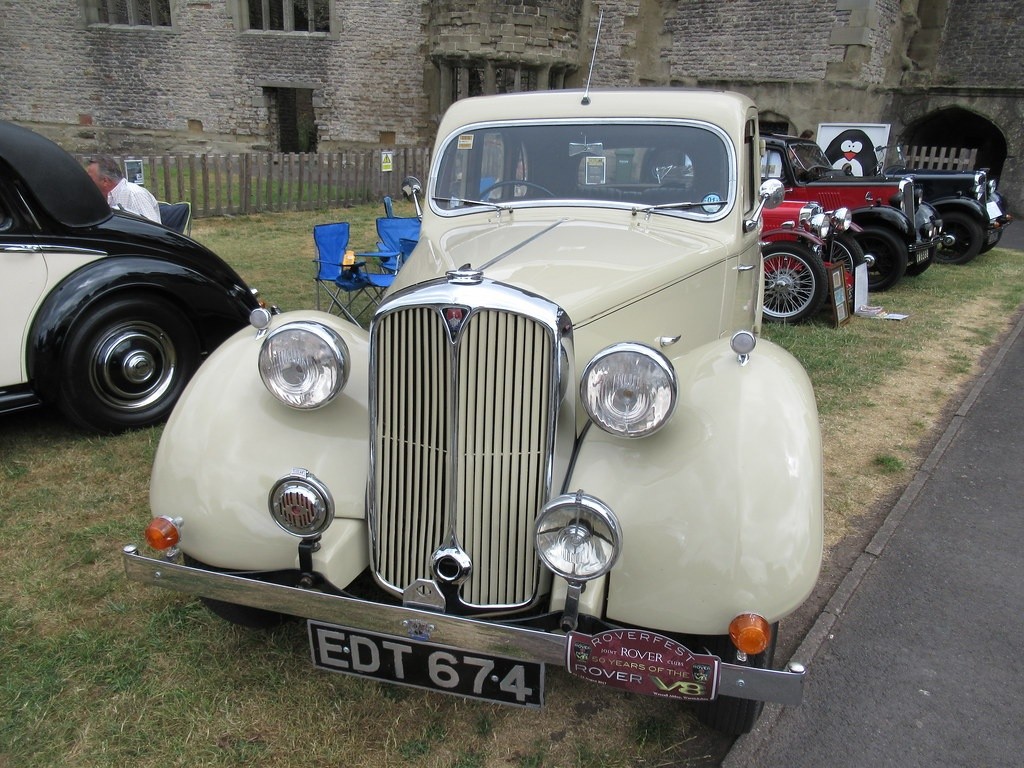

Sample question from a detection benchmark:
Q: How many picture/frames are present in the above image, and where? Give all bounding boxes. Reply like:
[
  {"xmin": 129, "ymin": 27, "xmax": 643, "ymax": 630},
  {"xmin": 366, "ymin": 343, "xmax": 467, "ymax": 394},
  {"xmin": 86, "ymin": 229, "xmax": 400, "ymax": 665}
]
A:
[
  {"xmin": 585, "ymin": 156, "xmax": 606, "ymax": 184},
  {"xmin": 827, "ymin": 260, "xmax": 851, "ymax": 330}
]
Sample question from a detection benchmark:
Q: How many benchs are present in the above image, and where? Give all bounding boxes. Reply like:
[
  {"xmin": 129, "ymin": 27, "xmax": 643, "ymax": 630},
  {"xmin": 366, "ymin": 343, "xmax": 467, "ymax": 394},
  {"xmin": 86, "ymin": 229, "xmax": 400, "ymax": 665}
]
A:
[{"xmin": 584, "ymin": 186, "xmax": 687, "ymax": 201}]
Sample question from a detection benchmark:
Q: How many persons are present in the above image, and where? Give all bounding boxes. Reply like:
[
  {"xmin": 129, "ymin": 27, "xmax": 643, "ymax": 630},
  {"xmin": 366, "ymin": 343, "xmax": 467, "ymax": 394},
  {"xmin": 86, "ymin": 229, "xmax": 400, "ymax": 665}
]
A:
[
  {"xmin": 789, "ymin": 130, "xmax": 814, "ymax": 176},
  {"xmin": 488, "ymin": 159, "xmax": 527, "ymax": 199},
  {"xmin": 87, "ymin": 155, "xmax": 162, "ymax": 225}
]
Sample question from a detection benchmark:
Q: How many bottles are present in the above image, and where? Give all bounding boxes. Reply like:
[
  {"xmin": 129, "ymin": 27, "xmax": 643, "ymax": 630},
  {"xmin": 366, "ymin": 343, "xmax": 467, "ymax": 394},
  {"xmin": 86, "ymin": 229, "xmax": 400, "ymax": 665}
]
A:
[{"xmin": 343, "ymin": 250, "xmax": 355, "ymax": 270}]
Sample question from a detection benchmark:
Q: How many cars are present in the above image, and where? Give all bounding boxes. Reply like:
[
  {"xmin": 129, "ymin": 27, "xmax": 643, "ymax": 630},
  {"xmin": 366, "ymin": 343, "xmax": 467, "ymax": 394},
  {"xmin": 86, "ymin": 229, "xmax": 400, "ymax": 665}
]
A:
[
  {"xmin": 638, "ymin": 134, "xmax": 1015, "ymax": 329},
  {"xmin": 124, "ymin": 87, "xmax": 826, "ymax": 740},
  {"xmin": 0, "ymin": 120, "xmax": 266, "ymax": 436}
]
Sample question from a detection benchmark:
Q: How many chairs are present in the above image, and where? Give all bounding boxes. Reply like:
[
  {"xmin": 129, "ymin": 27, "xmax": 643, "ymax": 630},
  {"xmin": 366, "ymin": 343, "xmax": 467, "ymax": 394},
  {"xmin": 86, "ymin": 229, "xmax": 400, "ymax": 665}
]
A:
[
  {"xmin": 527, "ymin": 157, "xmax": 577, "ymax": 194},
  {"xmin": 313, "ymin": 222, "xmax": 401, "ymax": 328},
  {"xmin": 375, "ymin": 197, "xmax": 420, "ymax": 300}
]
